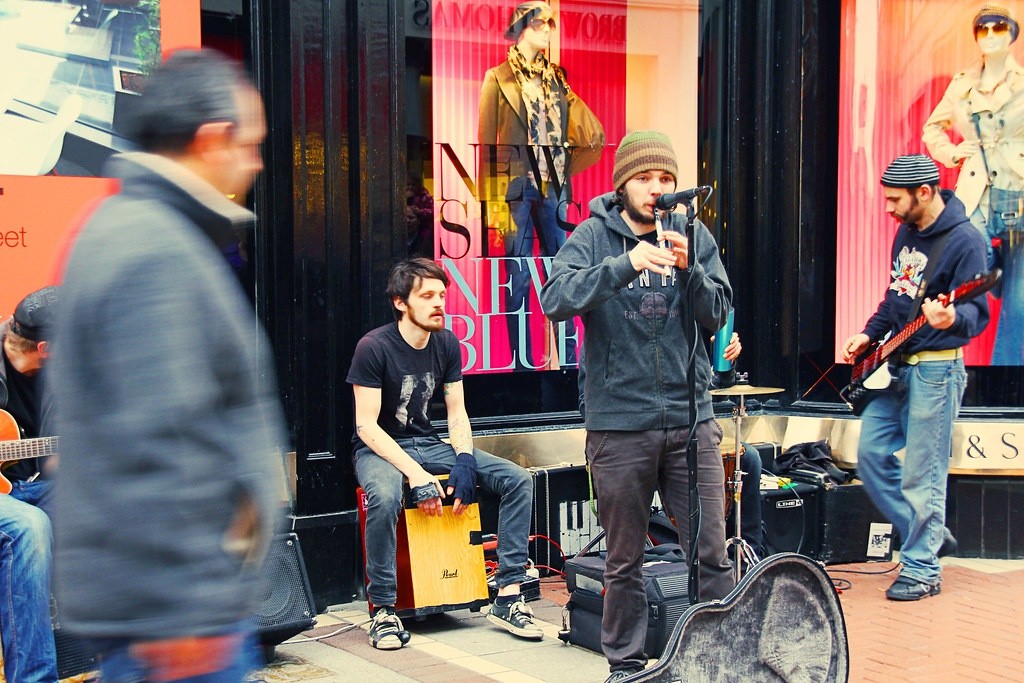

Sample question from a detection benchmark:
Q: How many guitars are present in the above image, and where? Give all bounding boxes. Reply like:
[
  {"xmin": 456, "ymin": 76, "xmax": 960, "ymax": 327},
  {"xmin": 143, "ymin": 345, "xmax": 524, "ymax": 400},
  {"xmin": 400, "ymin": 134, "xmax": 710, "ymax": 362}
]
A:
[
  {"xmin": 845, "ymin": 268, "xmax": 1004, "ymax": 407},
  {"xmin": 0, "ymin": 408, "xmax": 66, "ymax": 495}
]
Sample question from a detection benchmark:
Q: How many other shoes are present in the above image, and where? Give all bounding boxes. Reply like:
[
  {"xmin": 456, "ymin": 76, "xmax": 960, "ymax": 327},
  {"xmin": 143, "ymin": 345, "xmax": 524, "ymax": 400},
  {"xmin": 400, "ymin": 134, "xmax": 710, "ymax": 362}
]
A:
[{"xmin": 603, "ymin": 667, "xmax": 642, "ymax": 683}]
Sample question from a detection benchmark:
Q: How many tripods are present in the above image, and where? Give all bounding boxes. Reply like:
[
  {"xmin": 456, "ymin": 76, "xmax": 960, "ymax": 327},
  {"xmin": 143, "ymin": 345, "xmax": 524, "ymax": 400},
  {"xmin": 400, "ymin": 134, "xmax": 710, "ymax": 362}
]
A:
[{"xmin": 725, "ymin": 394, "xmax": 760, "ymax": 585}]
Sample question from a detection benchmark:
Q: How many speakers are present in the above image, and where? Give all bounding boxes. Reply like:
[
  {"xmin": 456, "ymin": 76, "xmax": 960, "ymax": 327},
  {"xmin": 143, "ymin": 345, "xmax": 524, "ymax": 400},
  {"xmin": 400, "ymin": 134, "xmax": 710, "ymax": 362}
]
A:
[
  {"xmin": 355, "ymin": 473, "xmax": 489, "ymax": 623},
  {"xmin": 254, "ymin": 532, "xmax": 318, "ymax": 649},
  {"xmin": 821, "ymin": 483, "xmax": 894, "ymax": 565},
  {"xmin": 760, "ymin": 480, "xmax": 820, "ymax": 563}
]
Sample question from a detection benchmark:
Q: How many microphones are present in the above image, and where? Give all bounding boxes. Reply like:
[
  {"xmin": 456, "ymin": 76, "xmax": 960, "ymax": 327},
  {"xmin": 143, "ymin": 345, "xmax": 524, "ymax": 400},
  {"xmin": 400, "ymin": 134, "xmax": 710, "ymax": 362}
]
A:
[{"xmin": 656, "ymin": 185, "xmax": 709, "ymax": 211}]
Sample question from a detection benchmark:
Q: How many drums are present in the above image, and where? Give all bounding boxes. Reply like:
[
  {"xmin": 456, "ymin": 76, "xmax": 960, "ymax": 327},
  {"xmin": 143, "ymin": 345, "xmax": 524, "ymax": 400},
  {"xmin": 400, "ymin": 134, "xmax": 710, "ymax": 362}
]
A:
[{"xmin": 658, "ymin": 437, "xmax": 747, "ymax": 527}]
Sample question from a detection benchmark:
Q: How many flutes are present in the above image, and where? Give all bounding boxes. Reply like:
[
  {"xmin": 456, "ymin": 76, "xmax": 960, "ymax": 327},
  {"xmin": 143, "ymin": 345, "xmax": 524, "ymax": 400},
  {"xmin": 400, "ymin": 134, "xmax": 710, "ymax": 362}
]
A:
[{"xmin": 653, "ymin": 207, "xmax": 671, "ymax": 280}]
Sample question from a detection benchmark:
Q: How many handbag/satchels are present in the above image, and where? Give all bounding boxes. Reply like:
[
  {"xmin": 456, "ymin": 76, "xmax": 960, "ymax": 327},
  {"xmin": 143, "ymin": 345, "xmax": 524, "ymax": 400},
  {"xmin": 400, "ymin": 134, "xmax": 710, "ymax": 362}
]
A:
[{"xmin": 986, "ymin": 188, "xmax": 1024, "ymax": 237}]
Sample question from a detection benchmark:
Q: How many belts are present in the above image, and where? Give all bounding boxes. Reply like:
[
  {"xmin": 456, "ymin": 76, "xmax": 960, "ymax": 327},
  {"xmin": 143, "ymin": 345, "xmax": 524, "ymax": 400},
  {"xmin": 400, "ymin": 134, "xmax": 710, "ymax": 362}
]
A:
[{"xmin": 899, "ymin": 347, "xmax": 962, "ymax": 365}]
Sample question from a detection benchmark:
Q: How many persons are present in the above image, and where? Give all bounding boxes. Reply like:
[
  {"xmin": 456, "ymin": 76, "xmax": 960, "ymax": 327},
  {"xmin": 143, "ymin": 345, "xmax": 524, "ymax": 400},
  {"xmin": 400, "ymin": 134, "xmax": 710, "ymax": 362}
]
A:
[
  {"xmin": 45, "ymin": 47, "xmax": 289, "ymax": 683},
  {"xmin": 840, "ymin": 151, "xmax": 990, "ymax": 600},
  {"xmin": 541, "ymin": 127, "xmax": 736, "ymax": 683},
  {"xmin": 475, "ymin": 0, "xmax": 578, "ymax": 372},
  {"xmin": 346, "ymin": 255, "xmax": 544, "ymax": 650},
  {"xmin": 921, "ymin": 3, "xmax": 1024, "ymax": 365},
  {"xmin": 579, "ymin": 332, "xmax": 764, "ymax": 557},
  {"xmin": 0, "ymin": 284, "xmax": 103, "ymax": 683}
]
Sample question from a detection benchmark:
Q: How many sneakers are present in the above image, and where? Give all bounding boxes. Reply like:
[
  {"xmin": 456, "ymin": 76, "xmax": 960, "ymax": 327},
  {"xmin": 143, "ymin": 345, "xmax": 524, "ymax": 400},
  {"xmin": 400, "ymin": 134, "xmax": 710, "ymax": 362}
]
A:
[
  {"xmin": 485, "ymin": 594, "xmax": 544, "ymax": 637},
  {"xmin": 936, "ymin": 537, "xmax": 957, "ymax": 558},
  {"xmin": 886, "ymin": 574, "xmax": 941, "ymax": 601},
  {"xmin": 368, "ymin": 606, "xmax": 404, "ymax": 649}
]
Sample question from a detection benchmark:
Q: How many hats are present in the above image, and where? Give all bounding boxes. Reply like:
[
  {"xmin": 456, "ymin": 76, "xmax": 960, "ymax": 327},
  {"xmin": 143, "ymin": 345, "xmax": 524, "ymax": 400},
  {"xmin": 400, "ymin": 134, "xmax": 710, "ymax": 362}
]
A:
[
  {"xmin": 11, "ymin": 286, "xmax": 59, "ymax": 341},
  {"xmin": 973, "ymin": 5, "xmax": 1020, "ymax": 46},
  {"xmin": 504, "ymin": 2, "xmax": 553, "ymax": 41},
  {"xmin": 613, "ymin": 131, "xmax": 678, "ymax": 192},
  {"xmin": 881, "ymin": 155, "xmax": 940, "ymax": 187}
]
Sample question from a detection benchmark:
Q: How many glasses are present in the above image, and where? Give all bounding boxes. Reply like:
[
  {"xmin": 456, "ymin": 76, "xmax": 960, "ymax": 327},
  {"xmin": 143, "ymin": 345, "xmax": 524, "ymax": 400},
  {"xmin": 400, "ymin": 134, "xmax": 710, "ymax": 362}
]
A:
[
  {"xmin": 974, "ymin": 23, "xmax": 1007, "ymax": 40},
  {"xmin": 530, "ymin": 17, "xmax": 556, "ymax": 33}
]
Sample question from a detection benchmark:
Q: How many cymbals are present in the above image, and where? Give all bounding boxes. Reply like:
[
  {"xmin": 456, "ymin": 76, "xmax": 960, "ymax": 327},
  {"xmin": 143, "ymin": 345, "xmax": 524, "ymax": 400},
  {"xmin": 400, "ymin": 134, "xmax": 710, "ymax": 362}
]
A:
[{"xmin": 707, "ymin": 384, "xmax": 787, "ymax": 396}]
